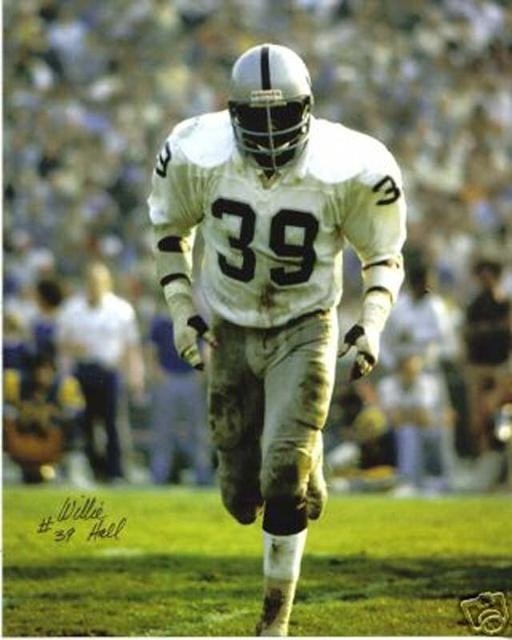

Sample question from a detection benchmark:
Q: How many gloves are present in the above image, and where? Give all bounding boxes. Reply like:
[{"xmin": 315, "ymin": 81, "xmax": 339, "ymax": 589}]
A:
[
  {"xmin": 338, "ymin": 288, "xmax": 392, "ymax": 380},
  {"xmin": 162, "ymin": 275, "xmax": 218, "ymax": 370}
]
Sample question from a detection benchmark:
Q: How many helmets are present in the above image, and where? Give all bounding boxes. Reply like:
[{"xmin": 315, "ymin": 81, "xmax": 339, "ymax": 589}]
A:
[{"xmin": 227, "ymin": 44, "xmax": 311, "ymax": 172}]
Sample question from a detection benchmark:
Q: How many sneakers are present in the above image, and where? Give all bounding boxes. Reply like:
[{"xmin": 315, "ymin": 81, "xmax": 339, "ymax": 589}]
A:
[{"xmin": 305, "ymin": 429, "xmax": 328, "ymax": 521}]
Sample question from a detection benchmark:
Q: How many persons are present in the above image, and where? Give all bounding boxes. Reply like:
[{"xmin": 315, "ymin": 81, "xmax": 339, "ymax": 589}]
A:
[
  {"xmin": 1, "ymin": 0, "xmax": 511, "ymax": 493},
  {"xmin": 147, "ymin": 41, "xmax": 407, "ymax": 635}
]
